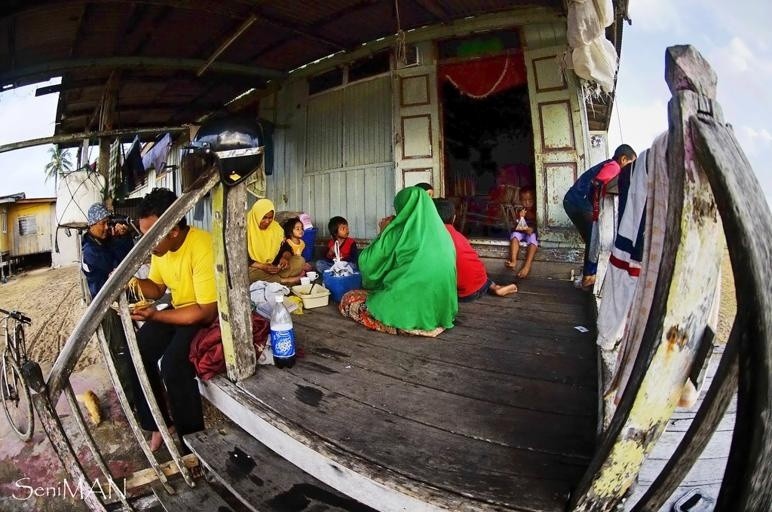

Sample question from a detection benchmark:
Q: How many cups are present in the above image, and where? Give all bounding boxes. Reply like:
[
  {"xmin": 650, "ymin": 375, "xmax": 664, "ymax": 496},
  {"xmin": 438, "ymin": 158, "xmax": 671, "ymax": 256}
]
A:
[
  {"xmin": 300, "ymin": 277, "xmax": 310, "ymax": 285},
  {"xmin": 306, "ymin": 271, "xmax": 319, "ymax": 281}
]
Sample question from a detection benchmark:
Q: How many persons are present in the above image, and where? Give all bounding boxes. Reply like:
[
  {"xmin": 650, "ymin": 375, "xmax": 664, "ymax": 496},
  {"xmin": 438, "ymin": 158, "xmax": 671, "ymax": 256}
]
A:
[
  {"xmin": 81, "ymin": 186, "xmax": 220, "ymax": 448},
  {"xmin": 562, "ymin": 143, "xmax": 638, "ymax": 285},
  {"xmin": 502, "ymin": 184, "xmax": 539, "ymax": 279},
  {"xmin": 247, "ymin": 184, "xmax": 518, "ymax": 337}
]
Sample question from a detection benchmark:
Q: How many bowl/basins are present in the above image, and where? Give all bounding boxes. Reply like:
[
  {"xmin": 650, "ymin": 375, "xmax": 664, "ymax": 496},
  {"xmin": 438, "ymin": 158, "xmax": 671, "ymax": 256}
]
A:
[{"xmin": 110, "ymin": 299, "xmax": 156, "ymax": 315}]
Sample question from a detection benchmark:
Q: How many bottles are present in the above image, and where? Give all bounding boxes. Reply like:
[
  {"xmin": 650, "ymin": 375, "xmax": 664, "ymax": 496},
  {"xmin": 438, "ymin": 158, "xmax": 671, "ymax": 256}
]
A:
[{"xmin": 270, "ymin": 296, "xmax": 297, "ymax": 368}]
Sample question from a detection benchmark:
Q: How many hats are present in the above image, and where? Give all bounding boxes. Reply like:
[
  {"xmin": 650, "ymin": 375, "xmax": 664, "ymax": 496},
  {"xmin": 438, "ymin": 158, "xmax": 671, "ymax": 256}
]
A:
[{"xmin": 87, "ymin": 202, "xmax": 112, "ymax": 226}]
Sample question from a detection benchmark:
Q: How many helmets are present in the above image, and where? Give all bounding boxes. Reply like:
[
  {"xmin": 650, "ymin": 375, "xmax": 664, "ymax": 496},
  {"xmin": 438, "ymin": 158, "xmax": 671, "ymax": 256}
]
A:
[{"xmin": 180, "ymin": 112, "xmax": 263, "ymax": 193}]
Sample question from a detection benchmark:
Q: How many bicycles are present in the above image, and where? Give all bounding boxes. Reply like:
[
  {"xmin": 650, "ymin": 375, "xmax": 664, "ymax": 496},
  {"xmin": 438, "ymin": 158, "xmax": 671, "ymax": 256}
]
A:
[{"xmin": 0, "ymin": 309, "xmax": 34, "ymax": 442}]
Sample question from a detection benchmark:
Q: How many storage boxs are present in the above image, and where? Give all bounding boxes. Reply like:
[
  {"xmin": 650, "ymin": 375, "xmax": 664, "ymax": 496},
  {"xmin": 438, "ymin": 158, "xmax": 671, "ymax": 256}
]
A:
[
  {"xmin": 323, "ymin": 272, "xmax": 362, "ymax": 304},
  {"xmin": 302, "ymin": 227, "xmax": 318, "ymax": 261}
]
